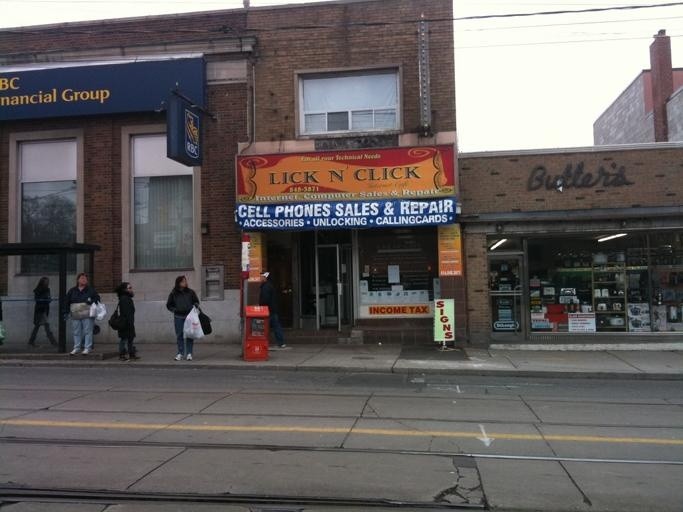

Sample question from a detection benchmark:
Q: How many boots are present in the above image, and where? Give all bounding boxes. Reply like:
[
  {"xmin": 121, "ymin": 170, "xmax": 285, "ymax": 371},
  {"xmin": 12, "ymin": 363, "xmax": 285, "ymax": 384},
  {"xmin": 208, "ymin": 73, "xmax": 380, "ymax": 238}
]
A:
[
  {"xmin": 118, "ymin": 351, "xmax": 128, "ymax": 362},
  {"xmin": 127, "ymin": 348, "xmax": 140, "ymax": 361}
]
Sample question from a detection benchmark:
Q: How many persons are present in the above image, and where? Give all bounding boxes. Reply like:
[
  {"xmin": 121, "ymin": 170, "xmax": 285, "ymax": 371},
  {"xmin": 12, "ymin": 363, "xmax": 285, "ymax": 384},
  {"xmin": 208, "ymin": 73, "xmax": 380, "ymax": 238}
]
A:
[
  {"xmin": 640, "ymin": 263, "xmax": 657, "ymax": 302},
  {"xmin": 260, "ymin": 272, "xmax": 288, "ymax": 350},
  {"xmin": 116, "ymin": 282, "xmax": 142, "ymax": 362},
  {"xmin": 66, "ymin": 273, "xmax": 101, "ymax": 356},
  {"xmin": 167, "ymin": 276, "xmax": 200, "ymax": 360},
  {"xmin": 30, "ymin": 280, "xmax": 57, "ymax": 345}
]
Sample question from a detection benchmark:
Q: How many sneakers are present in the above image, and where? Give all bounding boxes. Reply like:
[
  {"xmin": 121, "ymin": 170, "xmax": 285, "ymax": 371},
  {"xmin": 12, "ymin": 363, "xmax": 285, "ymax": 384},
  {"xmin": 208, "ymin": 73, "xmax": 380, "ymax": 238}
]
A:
[
  {"xmin": 185, "ymin": 353, "xmax": 193, "ymax": 361},
  {"xmin": 80, "ymin": 347, "xmax": 91, "ymax": 356},
  {"xmin": 69, "ymin": 349, "xmax": 79, "ymax": 357},
  {"xmin": 173, "ymin": 355, "xmax": 182, "ymax": 362}
]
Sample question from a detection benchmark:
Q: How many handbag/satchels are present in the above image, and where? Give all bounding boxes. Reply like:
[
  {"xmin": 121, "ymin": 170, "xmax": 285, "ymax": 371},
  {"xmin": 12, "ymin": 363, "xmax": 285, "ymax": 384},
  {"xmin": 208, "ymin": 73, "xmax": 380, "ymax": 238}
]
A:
[
  {"xmin": 90, "ymin": 300, "xmax": 107, "ymax": 321},
  {"xmin": 184, "ymin": 306, "xmax": 211, "ymax": 338}
]
